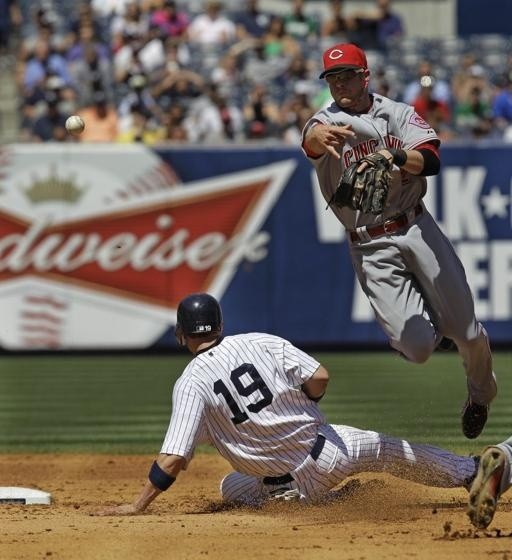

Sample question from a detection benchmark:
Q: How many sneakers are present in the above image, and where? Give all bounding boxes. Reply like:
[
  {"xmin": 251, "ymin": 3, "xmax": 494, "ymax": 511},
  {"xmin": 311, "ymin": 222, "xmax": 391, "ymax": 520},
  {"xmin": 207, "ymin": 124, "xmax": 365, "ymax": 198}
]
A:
[
  {"xmin": 466, "ymin": 448, "xmax": 504, "ymax": 530},
  {"xmin": 461, "ymin": 400, "xmax": 488, "ymax": 440}
]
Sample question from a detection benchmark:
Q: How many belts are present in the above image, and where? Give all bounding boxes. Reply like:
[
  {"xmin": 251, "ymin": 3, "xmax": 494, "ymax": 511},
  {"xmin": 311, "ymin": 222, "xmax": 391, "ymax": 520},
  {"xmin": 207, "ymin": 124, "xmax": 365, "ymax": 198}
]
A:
[
  {"xmin": 262, "ymin": 433, "xmax": 325, "ymax": 488},
  {"xmin": 348, "ymin": 203, "xmax": 423, "ymax": 246}
]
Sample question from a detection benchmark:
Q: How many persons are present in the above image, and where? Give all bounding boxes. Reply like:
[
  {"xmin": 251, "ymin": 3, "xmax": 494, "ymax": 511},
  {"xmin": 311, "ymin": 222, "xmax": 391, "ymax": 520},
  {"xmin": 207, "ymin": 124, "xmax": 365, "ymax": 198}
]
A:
[
  {"xmin": 94, "ymin": 290, "xmax": 483, "ymax": 516},
  {"xmin": 1, "ymin": 0, "xmax": 512, "ymax": 142},
  {"xmin": 466, "ymin": 433, "xmax": 512, "ymax": 528},
  {"xmin": 300, "ymin": 42, "xmax": 499, "ymax": 439}
]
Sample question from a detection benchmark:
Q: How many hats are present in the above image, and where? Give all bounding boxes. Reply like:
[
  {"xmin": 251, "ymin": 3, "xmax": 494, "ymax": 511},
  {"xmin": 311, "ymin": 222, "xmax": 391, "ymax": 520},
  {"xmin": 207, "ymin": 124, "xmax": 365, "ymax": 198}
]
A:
[{"xmin": 319, "ymin": 41, "xmax": 368, "ymax": 84}]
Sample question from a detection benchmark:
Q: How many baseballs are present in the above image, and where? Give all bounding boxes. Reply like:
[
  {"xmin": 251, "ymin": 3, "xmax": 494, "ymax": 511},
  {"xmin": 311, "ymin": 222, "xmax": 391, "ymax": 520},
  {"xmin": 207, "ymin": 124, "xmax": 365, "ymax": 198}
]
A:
[{"xmin": 66, "ymin": 116, "xmax": 84, "ymax": 135}]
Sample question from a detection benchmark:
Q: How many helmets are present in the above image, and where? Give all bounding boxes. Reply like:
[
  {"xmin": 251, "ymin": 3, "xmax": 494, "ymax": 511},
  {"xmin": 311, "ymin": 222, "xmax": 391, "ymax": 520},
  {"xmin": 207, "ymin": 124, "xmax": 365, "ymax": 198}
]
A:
[{"xmin": 176, "ymin": 291, "xmax": 224, "ymax": 337}]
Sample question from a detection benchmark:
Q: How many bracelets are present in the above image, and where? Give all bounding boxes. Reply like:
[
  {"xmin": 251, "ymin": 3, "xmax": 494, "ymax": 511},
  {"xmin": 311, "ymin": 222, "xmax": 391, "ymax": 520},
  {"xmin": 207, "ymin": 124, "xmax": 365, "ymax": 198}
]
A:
[
  {"xmin": 301, "ymin": 384, "xmax": 326, "ymax": 404},
  {"xmin": 385, "ymin": 148, "xmax": 408, "ymax": 167},
  {"xmin": 147, "ymin": 458, "xmax": 175, "ymax": 492}
]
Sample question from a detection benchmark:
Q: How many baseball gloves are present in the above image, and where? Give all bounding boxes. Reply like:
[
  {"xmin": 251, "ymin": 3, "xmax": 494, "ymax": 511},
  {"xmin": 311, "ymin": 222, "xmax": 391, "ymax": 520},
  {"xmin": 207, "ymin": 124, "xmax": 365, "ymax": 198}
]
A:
[{"xmin": 326, "ymin": 153, "xmax": 392, "ymax": 214}]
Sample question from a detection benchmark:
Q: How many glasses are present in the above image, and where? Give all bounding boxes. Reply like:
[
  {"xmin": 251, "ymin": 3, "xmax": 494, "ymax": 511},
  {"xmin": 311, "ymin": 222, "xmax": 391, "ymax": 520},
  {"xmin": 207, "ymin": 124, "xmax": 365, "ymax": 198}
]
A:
[{"xmin": 326, "ymin": 71, "xmax": 363, "ymax": 84}]
